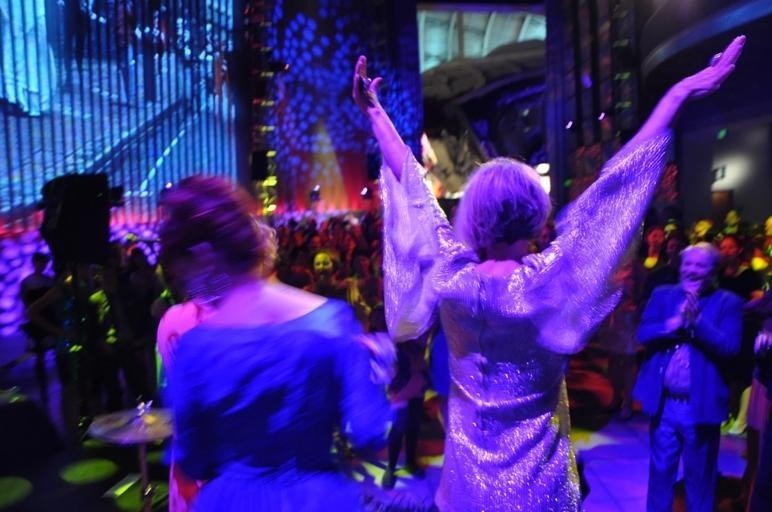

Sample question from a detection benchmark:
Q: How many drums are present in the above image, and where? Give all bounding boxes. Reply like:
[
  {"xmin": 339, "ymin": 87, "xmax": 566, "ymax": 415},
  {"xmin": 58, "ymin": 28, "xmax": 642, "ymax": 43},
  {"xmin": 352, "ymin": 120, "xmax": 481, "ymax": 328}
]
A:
[
  {"xmin": 57, "ymin": 458, "xmax": 120, "ymax": 512},
  {"xmin": 102, "ymin": 472, "xmax": 169, "ymax": 512}
]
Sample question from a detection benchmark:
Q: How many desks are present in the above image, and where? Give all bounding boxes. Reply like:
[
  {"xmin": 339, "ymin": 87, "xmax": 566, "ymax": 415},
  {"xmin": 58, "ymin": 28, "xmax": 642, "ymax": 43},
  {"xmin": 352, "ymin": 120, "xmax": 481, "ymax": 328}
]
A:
[{"xmin": 89, "ymin": 408, "xmax": 174, "ymax": 511}]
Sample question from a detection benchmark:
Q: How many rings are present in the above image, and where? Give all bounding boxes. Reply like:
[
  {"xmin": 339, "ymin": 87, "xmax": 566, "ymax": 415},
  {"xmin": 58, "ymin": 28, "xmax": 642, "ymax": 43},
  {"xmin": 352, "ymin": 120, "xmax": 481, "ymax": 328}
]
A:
[{"xmin": 709, "ymin": 53, "xmax": 723, "ymax": 66}]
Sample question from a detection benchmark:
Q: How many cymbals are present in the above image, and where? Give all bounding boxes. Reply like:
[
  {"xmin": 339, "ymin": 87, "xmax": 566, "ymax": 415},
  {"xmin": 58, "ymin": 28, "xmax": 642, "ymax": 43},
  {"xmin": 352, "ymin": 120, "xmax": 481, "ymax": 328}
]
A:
[{"xmin": 88, "ymin": 408, "xmax": 174, "ymax": 445}]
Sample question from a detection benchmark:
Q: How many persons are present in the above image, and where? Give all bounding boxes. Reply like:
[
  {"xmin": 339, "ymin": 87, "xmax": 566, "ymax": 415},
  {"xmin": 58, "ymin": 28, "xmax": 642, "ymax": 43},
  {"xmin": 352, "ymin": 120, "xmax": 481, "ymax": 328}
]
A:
[
  {"xmin": 0, "ymin": 202, "xmax": 772, "ymax": 512},
  {"xmin": 352, "ymin": 32, "xmax": 752, "ymax": 510},
  {"xmin": 152, "ymin": 170, "xmax": 420, "ymax": 511}
]
